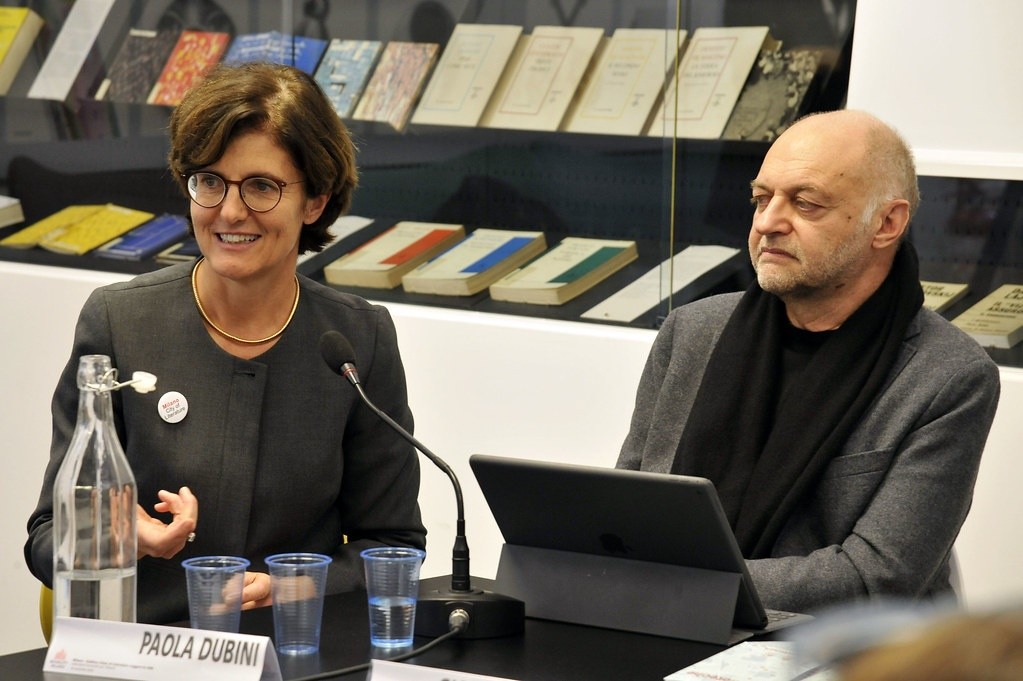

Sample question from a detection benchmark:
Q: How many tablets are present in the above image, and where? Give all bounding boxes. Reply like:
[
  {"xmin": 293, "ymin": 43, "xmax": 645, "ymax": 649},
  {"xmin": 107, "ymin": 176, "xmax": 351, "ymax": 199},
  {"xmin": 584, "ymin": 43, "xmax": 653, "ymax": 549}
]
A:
[{"xmin": 470, "ymin": 454, "xmax": 768, "ymax": 631}]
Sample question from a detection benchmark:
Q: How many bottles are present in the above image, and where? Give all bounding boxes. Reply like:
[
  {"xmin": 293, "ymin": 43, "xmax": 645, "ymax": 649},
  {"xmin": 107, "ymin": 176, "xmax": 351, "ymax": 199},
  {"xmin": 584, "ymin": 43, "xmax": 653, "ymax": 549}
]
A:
[{"xmin": 52, "ymin": 354, "xmax": 138, "ymax": 627}]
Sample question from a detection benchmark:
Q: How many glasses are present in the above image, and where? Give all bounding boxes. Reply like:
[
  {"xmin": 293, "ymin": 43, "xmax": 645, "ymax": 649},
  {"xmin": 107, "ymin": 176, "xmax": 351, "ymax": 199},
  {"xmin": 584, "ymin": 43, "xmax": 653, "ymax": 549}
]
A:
[{"xmin": 180, "ymin": 171, "xmax": 305, "ymax": 213}]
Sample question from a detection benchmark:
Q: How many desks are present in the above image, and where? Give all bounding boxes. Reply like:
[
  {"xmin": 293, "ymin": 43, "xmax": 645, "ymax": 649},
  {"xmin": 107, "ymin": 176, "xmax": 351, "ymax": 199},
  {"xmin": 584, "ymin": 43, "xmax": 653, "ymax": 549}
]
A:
[{"xmin": 0, "ymin": 567, "xmax": 1023, "ymax": 681}]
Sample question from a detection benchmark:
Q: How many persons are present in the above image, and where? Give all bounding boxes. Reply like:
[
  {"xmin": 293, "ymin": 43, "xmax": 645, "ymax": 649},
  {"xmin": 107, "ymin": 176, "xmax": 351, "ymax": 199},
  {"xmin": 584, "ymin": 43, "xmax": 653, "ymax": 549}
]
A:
[
  {"xmin": 616, "ymin": 110, "xmax": 1001, "ymax": 619},
  {"xmin": 23, "ymin": 61, "xmax": 428, "ymax": 625}
]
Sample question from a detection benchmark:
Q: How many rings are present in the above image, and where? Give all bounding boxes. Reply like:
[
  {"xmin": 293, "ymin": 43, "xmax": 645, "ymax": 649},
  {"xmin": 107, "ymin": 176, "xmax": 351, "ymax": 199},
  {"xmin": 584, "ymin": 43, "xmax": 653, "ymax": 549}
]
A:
[{"xmin": 187, "ymin": 532, "xmax": 196, "ymax": 542}]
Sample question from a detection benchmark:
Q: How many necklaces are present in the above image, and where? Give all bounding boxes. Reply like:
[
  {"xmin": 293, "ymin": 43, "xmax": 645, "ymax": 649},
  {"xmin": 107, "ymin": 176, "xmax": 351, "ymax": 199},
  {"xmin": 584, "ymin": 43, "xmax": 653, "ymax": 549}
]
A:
[{"xmin": 192, "ymin": 258, "xmax": 300, "ymax": 343}]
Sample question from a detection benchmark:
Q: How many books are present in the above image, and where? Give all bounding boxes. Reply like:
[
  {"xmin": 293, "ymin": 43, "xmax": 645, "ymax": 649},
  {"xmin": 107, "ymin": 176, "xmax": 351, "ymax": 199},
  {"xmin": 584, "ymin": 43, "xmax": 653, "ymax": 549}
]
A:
[
  {"xmin": 951, "ymin": 284, "xmax": 1023, "ymax": 348},
  {"xmin": 98, "ymin": 23, "xmax": 783, "ymax": 139},
  {"xmin": 0, "ymin": 7, "xmax": 43, "ymax": 96},
  {"xmin": 402, "ymin": 228, "xmax": 547, "ymax": 296},
  {"xmin": 489, "ymin": 236, "xmax": 638, "ymax": 305},
  {"xmin": 920, "ymin": 280, "xmax": 968, "ymax": 314},
  {"xmin": 0, "ymin": 195, "xmax": 202, "ymax": 264},
  {"xmin": 324, "ymin": 222, "xmax": 464, "ymax": 288}
]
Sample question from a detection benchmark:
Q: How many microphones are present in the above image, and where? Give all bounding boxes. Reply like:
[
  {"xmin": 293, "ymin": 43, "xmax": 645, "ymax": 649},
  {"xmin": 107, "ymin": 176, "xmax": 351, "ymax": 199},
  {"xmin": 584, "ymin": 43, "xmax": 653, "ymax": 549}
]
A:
[{"xmin": 321, "ymin": 331, "xmax": 525, "ymax": 639}]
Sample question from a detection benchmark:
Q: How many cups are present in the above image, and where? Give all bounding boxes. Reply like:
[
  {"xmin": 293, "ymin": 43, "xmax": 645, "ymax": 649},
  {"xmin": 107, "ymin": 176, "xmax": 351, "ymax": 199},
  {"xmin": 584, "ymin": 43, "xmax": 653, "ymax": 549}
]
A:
[
  {"xmin": 182, "ymin": 556, "xmax": 251, "ymax": 633},
  {"xmin": 265, "ymin": 553, "xmax": 333, "ymax": 656},
  {"xmin": 360, "ymin": 547, "xmax": 426, "ymax": 646}
]
